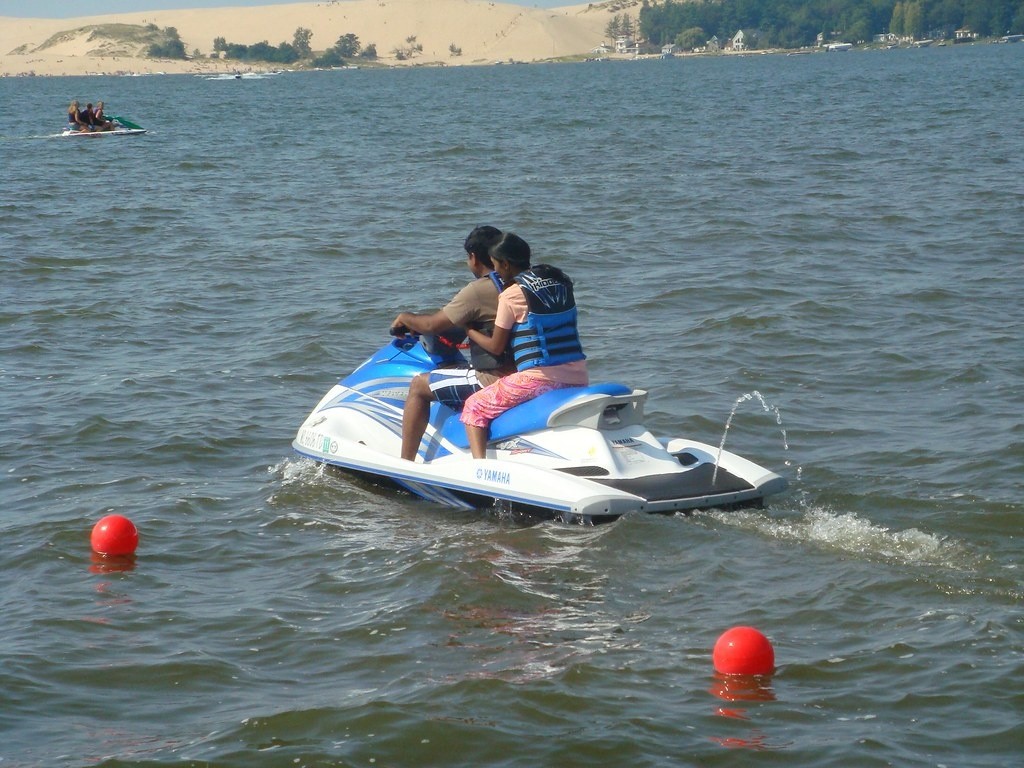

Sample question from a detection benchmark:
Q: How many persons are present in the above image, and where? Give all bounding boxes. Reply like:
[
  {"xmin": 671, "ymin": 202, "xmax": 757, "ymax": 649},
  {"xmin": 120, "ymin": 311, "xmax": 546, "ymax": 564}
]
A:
[
  {"xmin": 460, "ymin": 231, "xmax": 589, "ymax": 459},
  {"xmin": 68, "ymin": 100, "xmax": 114, "ymax": 132},
  {"xmin": 391, "ymin": 226, "xmax": 517, "ymax": 462}
]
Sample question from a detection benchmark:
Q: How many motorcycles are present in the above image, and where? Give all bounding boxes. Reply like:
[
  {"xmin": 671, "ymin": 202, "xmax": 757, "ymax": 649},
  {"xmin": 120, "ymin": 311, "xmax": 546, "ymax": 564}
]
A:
[
  {"xmin": 61, "ymin": 115, "xmax": 148, "ymax": 137},
  {"xmin": 290, "ymin": 325, "xmax": 789, "ymax": 526}
]
